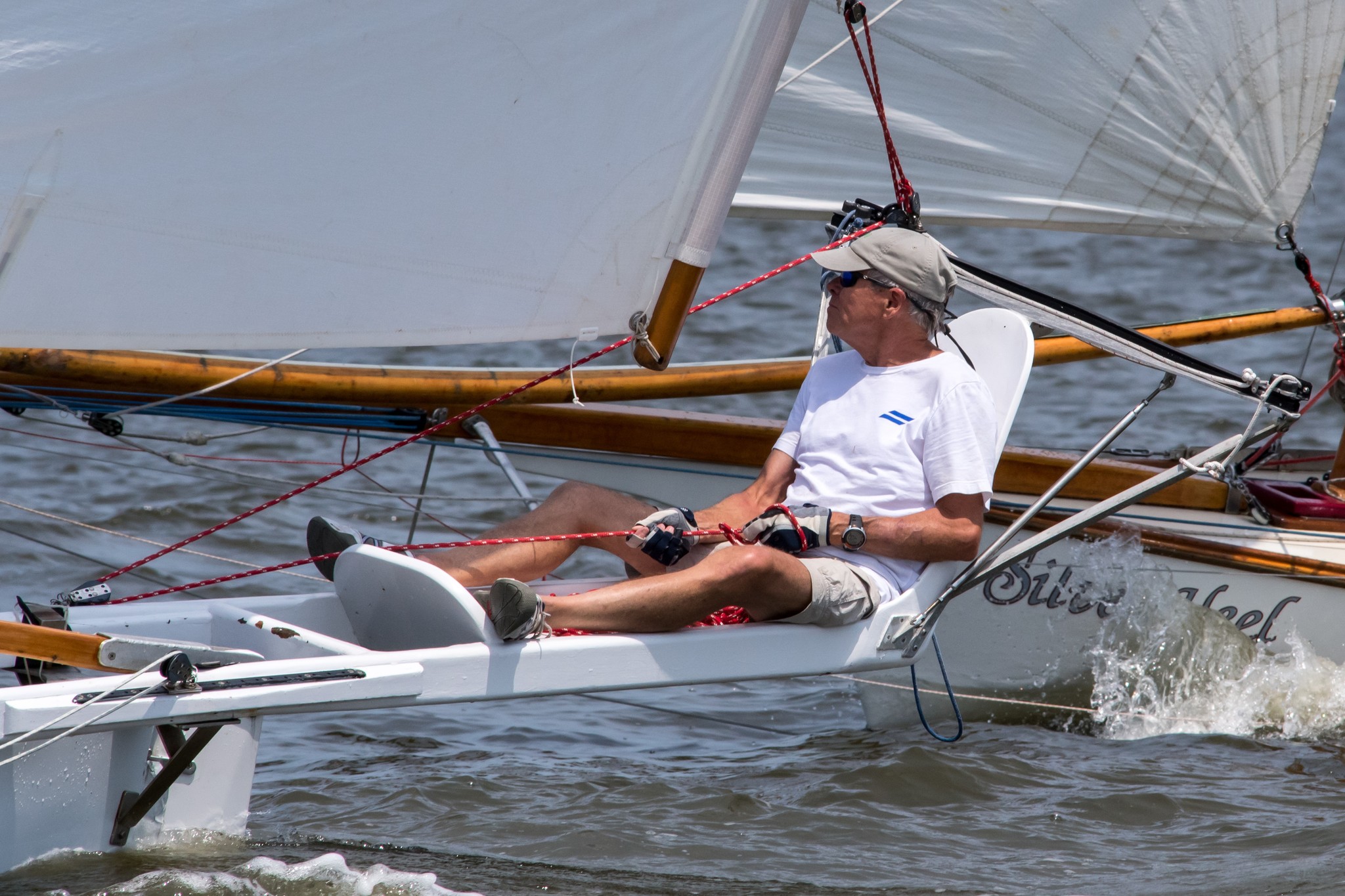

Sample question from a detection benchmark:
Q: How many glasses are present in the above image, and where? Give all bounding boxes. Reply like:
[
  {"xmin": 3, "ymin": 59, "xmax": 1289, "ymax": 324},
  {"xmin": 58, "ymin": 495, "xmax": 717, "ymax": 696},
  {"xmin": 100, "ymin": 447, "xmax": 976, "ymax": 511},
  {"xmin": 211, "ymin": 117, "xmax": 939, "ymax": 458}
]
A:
[{"xmin": 840, "ymin": 270, "xmax": 922, "ymax": 309}]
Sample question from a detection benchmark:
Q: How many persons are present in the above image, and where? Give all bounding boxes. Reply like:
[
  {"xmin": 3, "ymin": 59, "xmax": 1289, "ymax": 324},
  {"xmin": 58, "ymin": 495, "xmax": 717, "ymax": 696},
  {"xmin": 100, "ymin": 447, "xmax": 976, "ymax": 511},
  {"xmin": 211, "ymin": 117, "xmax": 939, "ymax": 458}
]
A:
[{"xmin": 307, "ymin": 228, "xmax": 993, "ymax": 641}]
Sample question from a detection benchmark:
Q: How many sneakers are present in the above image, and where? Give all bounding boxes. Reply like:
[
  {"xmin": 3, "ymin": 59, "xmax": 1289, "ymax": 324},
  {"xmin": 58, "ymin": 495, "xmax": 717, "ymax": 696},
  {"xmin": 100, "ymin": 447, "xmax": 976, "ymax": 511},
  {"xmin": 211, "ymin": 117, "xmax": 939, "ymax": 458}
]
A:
[
  {"xmin": 473, "ymin": 577, "xmax": 552, "ymax": 641},
  {"xmin": 306, "ymin": 516, "xmax": 415, "ymax": 582}
]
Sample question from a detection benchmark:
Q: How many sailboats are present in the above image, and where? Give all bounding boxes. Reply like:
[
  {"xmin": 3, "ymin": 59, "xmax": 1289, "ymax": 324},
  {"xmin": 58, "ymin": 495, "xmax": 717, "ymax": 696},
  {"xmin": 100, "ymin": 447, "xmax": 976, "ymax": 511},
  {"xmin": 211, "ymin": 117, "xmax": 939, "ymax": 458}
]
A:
[{"xmin": 0, "ymin": 1, "xmax": 1345, "ymax": 846}]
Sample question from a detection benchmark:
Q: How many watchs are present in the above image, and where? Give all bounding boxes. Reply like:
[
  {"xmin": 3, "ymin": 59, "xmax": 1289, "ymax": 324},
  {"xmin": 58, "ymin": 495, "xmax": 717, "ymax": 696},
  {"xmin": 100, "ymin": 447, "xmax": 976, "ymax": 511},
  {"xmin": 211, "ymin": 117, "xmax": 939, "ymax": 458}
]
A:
[{"xmin": 841, "ymin": 513, "xmax": 866, "ymax": 552}]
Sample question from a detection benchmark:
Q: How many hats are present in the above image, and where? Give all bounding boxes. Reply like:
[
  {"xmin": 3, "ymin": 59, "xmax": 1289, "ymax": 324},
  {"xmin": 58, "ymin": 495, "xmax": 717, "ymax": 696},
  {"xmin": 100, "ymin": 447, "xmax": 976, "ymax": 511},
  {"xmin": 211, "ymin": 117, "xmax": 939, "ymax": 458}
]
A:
[{"xmin": 810, "ymin": 227, "xmax": 958, "ymax": 303}]
